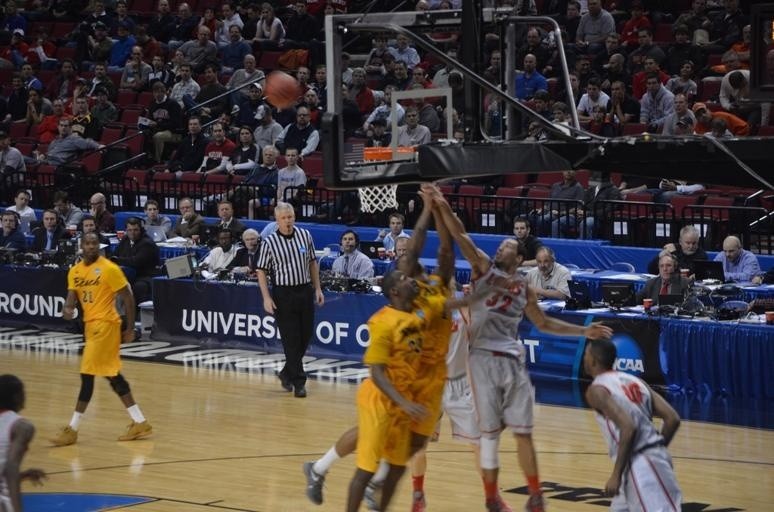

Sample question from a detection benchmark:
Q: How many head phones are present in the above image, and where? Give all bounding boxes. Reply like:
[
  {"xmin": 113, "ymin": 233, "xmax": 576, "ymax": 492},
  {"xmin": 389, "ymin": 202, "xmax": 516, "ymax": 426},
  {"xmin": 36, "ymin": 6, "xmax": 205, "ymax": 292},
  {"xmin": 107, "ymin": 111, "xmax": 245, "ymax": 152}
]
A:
[
  {"xmin": 239, "ymin": 226, "xmax": 263, "ymax": 246},
  {"xmin": 339, "ymin": 230, "xmax": 359, "ymax": 248},
  {"xmin": 51, "ymin": 209, "xmax": 62, "ymax": 228},
  {"xmin": 9, "ymin": 210, "xmax": 21, "ymax": 226},
  {"xmin": 124, "ymin": 216, "xmax": 146, "ymax": 234}
]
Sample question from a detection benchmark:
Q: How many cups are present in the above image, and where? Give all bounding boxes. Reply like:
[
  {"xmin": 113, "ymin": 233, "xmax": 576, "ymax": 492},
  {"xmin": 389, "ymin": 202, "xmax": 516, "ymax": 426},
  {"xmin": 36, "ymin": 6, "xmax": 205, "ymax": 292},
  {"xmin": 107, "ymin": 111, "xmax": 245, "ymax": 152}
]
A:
[
  {"xmin": 117, "ymin": 231, "xmax": 125, "ymax": 240},
  {"xmin": 68, "ymin": 224, "xmax": 77, "ymax": 237},
  {"xmin": 644, "ymin": 299, "xmax": 653, "ymax": 312},
  {"xmin": 193, "ymin": 234, "xmax": 200, "ymax": 246},
  {"xmin": 390, "ymin": 253, "xmax": 395, "ymax": 261},
  {"xmin": 765, "ymin": 312, "xmax": 774, "ymax": 325},
  {"xmin": 378, "ymin": 247, "xmax": 386, "ymax": 260},
  {"xmin": 324, "ymin": 248, "xmax": 330, "ymax": 256},
  {"xmin": 463, "ymin": 285, "xmax": 470, "ymax": 295},
  {"xmin": 680, "ymin": 269, "xmax": 689, "ymax": 279}
]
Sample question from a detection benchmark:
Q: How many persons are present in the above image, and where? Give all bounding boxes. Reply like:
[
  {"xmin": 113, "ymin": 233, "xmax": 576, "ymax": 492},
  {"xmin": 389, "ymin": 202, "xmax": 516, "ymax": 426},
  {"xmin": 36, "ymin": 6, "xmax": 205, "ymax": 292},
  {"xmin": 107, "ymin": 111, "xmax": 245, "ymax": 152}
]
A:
[
  {"xmin": 302, "ymin": 182, "xmax": 614, "ymax": 511},
  {"xmin": 255, "ymin": 202, "xmax": 326, "ymax": 398},
  {"xmin": 583, "ymin": 337, "xmax": 683, "ymax": 512},
  {"xmin": 48, "ymin": 233, "xmax": 153, "ymax": 446},
  {"xmin": 0, "ymin": 373, "xmax": 49, "ymax": 512},
  {"xmin": 0, "ymin": 0, "xmax": 774, "ymax": 312}
]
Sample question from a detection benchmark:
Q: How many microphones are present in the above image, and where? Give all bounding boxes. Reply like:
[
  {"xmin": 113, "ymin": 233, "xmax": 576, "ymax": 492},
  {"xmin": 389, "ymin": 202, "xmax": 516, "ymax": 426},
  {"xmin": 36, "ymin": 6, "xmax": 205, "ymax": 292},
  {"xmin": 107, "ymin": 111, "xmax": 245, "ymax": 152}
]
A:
[
  {"xmin": 252, "ymin": 244, "xmax": 258, "ymax": 246},
  {"xmin": 349, "ymin": 244, "xmax": 358, "ymax": 248},
  {"xmin": 11, "ymin": 227, "xmax": 18, "ymax": 230},
  {"xmin": 50, "ymin": 224, "xmax": 60, "ymax": 227}
]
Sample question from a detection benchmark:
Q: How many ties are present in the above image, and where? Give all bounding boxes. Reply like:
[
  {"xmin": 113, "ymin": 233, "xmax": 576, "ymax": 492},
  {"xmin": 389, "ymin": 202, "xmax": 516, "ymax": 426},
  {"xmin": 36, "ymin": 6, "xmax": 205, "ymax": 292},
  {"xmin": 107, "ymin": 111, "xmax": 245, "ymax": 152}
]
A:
[
  {"xmin": 660, "ymin": 282, "xmax": 670, "ymax": 294},
  {"xmin": 46, "ymin": 233, "xmax": 53, "ymax": 250},
  {"xmin": 344, "ymin": 256, "xmax": 349, "ymax": 274}
]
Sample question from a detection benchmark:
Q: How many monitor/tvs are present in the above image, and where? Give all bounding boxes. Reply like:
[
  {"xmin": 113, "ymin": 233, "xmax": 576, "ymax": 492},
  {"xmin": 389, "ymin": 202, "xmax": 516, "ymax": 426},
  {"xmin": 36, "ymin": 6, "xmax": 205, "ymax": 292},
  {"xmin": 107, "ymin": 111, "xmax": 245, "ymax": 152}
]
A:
[
  {"xmin": 601, "ymin": 280, "xmax": 636, "ymax": 313},
  {"xmin": 567, "ymin": 279, "xmax": 591, "ymax": 308}
]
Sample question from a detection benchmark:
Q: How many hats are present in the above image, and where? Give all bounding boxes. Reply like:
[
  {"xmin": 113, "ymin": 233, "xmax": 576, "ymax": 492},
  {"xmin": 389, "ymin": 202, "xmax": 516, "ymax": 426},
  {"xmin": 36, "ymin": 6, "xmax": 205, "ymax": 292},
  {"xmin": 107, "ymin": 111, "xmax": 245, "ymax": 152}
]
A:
[
  {"xmin": 250, "ymin": 83, "xmax": 262, "ymax": 92},
  {"xmin": 255, "ymin": 106, "xmax": 270, "ymax": 119},
  {"xmin": 692, "ymin": 103, "xmax": 707, "ymax": 112},
  {"xmin": 370, "ymin": 115, "xmax": 388, "ymax": 127},
  {"xmin": 13, "ymin": 28, "xmax": 26, "ymax": 37}
]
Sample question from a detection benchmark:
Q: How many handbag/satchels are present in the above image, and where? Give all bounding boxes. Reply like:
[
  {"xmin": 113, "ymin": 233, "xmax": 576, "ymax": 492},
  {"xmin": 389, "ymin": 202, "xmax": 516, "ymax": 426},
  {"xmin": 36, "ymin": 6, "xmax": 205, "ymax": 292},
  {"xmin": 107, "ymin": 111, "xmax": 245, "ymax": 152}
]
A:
[{"xmin": 205, "ymin": 158, "xmax": 222, "ymax": 170}]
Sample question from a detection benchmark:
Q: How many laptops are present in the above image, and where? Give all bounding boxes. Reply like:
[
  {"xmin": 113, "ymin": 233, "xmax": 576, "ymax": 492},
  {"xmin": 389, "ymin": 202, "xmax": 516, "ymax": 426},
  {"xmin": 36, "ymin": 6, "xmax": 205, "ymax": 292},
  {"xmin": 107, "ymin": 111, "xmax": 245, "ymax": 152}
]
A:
[
  {"xmin": 659, "ymin": 293, "xmax": 684, "ymax": 307},
  {"xmin": 144, "ymin": 225, "xmax": 167, "ymax": 242},
  {"xmin": 692, "ymin": 258, "xmax": 735, "ymax": 284},
  {"xmin": 360, "ymin": 240, "xmax": 384, "ymax": 260}
]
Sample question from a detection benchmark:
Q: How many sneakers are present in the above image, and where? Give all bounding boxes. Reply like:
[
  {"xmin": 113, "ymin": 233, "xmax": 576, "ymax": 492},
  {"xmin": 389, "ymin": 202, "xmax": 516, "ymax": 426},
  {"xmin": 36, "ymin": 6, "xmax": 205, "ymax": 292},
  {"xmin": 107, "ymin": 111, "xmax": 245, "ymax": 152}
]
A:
[
  {"xmin": 48, "ymin": 424, "xmax": 77, "ymax": 446},
  {"xmin": 295, "ymin": 384, "xmax": 307, "ymax": 397},
  {"xmin": 119, "ymin": 419, "xmax": 152, "ymax": 440},
  {"xmin": 278, "ymin": 372, "xmax": 293, "ymax": 392},
  {"xmin": 302, "ymin": 461, "xmax": 545, "ymax": 512}
]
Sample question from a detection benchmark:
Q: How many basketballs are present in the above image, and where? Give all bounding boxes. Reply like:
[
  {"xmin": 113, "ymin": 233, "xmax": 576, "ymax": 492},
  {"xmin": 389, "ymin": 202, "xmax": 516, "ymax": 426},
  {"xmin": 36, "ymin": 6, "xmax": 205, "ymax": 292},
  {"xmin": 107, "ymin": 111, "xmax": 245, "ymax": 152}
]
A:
[{"xmin": 265, "ymin": 72, "xmax": 299, "ymax": 107}]
